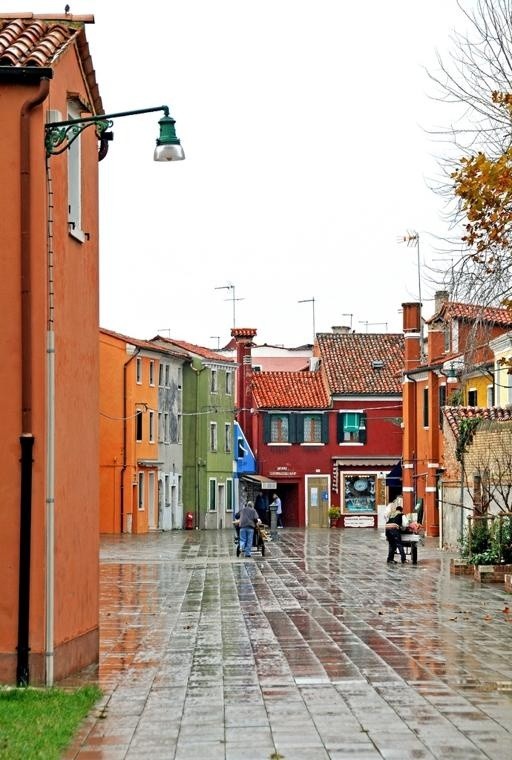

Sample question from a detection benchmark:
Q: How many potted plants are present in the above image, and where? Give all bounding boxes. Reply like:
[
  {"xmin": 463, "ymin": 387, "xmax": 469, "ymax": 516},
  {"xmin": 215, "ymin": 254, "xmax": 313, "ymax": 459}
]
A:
[
  {"xmin": 328, "ymin": 504, "xmax": 341, "ymax": 528},
  {"xmin": 449, "ymin": 511, "xmax": 512, "ymax": 594},
  {"xmin": 455, "ymin": 418, "xmax": 482, "ymax": 462}
]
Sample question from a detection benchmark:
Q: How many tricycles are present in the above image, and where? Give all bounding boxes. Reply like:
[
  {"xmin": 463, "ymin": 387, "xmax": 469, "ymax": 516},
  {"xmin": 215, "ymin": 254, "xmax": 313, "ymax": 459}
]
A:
[{"xmin": 386, "ymin": 526, "xmax": 422, "ymax": 566}]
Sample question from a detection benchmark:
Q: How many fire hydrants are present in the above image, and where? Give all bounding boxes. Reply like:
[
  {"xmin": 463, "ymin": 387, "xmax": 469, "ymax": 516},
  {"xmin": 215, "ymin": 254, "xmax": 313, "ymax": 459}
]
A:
[{"xmin": 184, "ymin": 511, "xmax": 195, "ymax": 531}]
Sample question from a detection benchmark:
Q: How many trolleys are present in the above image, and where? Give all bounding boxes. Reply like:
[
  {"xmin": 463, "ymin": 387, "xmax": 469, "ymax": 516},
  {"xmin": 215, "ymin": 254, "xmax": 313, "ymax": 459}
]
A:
[{"xmin": 232, "ymin": 520, "xmax": 269, "ymax": 559}]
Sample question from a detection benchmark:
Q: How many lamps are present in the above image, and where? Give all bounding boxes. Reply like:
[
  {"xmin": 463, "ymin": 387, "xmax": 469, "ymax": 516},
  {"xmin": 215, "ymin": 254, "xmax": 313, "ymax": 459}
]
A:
[
  {"xmin": 443, "ymin": 357, "xmax": 512, "ymax": 388},
  {"xmin": 46, "ymin": 104, "xmax": 186, "ymax": 163}
]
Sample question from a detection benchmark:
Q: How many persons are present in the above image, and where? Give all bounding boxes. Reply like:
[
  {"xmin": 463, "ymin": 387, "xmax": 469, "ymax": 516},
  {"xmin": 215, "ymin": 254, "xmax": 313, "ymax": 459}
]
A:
[
  {"xmin": 385, "ymin": 506, "xmax": 409, "ymax": 563},
  {"xmin": 269, "ymin": 494, "xmax": 284, "ymax": 528},
  {"xmin": 255, "ymin": 494, "xmax": 264, "ymax": 525},
  {"xmin": 235, "ymin": 501, "xmax": 262, "ymax": 557},
  {"xmin": 262, "ymin": 491, "xmax": 270, "ymax": 526}
]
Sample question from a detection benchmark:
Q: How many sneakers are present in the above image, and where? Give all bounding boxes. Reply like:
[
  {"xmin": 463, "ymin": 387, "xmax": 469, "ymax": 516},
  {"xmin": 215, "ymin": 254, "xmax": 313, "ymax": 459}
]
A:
[{"xmin": 387, "ymin": 559, "xmax": 409, "ymax": 565}]
